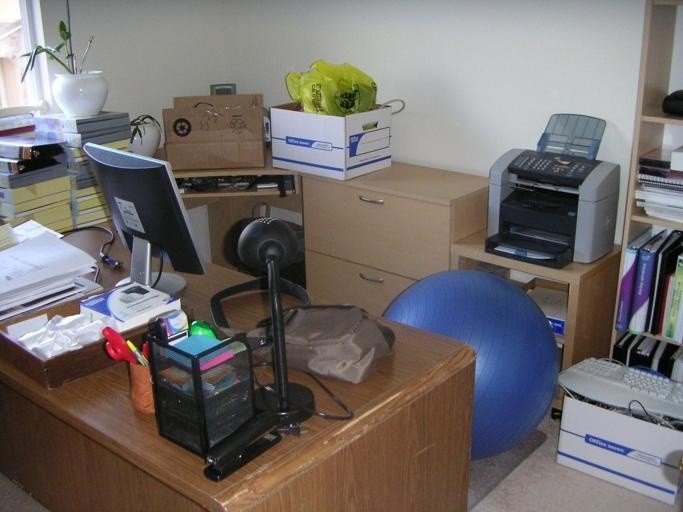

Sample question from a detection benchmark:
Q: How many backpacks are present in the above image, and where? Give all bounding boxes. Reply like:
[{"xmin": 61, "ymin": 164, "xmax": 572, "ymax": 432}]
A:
[{"xmin": 208, "ymin": 272, "xmax": 395, "ymax": 385}]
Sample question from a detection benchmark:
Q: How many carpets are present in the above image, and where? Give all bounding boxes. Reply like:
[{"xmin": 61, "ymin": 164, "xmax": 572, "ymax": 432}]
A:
[{"xmin": 469, "ymin": 429, "xmax": 548, "ymax": 512}]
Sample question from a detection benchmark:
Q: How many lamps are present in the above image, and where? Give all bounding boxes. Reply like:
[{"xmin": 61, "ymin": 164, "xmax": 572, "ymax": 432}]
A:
[{"xmin": 238, "ymin": 215, "xmax": 315, "ymax": 427}]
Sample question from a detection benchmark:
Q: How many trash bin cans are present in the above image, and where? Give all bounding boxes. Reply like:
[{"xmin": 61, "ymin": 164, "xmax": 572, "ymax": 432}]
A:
[{"xmin": 225, "ymin": 217, "xmax": 305, "ymax": 288}]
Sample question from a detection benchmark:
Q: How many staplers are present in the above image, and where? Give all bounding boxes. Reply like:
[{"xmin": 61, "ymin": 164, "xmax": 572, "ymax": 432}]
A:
[{"xmin": 204, "ymin": 411, "xmax": 281, "ymax": 483}]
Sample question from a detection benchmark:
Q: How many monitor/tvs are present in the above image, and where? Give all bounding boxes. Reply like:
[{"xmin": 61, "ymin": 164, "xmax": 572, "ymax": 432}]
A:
[{"xmin": 82, "ymin": 141, "xmax": 207, "ymax": 297}]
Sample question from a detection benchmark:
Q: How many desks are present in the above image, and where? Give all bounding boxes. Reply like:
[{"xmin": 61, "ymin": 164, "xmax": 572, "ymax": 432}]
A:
[
  {"xmin": 0, "ymin": 217, "xmax": 476, "ymax": 512},
  {"xmin": 153, "ymin": 148, "xmax": 303, "ymax": 179}
]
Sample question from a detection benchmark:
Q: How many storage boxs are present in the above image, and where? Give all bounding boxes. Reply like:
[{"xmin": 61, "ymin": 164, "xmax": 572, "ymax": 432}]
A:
[
  {"xmin": 271, "ymin": 102, "xmax": 392, "ymax": 180},
  {"xmin": 163, "ymin": 94, "xmax": 264, "ymax": 171},
  {"xmin": 556, "ymin": 392, "xmax": 682, "ymax": 507}
]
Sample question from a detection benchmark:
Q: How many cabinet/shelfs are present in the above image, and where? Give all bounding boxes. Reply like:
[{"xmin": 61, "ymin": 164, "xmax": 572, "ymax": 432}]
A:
[
  {"xmin": 452, "ymin": 228, "xmax": 621, "ymax": 420},
  {"xmin": 608, "ymin": 0, "xmax": 683, "ymax": 381},
  {"xmin": 304, "ymin": 159, "xmax": 489, "ymax": 320}
]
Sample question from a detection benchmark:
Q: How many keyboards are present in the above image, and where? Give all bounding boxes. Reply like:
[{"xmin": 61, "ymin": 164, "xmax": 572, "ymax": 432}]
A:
[{"xmin": 558, "ymin": 357, "xmax": 683, "ymax": 420}]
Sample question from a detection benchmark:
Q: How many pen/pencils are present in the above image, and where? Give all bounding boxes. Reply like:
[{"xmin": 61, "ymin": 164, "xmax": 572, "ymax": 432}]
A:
[{"xmin": 126, "ymin": 339, "xmax": 151, "ymax": 366}]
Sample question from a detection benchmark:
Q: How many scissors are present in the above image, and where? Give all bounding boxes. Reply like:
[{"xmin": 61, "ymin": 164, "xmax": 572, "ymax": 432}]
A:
[{"xmin": 102, "ymin": 327, "xmax": 138, "ymax": 364}]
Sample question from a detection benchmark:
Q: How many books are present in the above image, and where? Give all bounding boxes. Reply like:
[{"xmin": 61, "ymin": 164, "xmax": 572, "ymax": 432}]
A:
[
  {"xmin": 610, "ymin": 141, "xmax": 682, "ymax": 386},
  {"xmin": 0, "ymin": 107, "xmax": 134, "ymax": 326}
]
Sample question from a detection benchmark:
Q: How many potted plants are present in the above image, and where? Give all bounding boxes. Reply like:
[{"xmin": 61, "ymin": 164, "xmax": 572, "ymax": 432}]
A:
[
  {"xmin": 128, "ymin": 113, "xmax": 163, "ymax": 157},
  {"xmin": 19, "ymin": 20, "xmax": 109, "ymax": 116}
]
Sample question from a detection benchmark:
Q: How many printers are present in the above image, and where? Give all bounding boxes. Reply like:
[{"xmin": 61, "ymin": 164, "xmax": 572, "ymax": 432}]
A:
[{"xmin": 485, "ymin": 114, "xmax": 621, "ymax": 267}]
